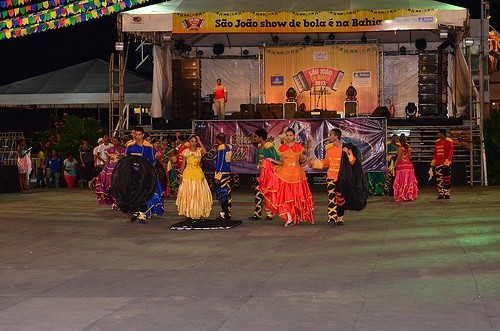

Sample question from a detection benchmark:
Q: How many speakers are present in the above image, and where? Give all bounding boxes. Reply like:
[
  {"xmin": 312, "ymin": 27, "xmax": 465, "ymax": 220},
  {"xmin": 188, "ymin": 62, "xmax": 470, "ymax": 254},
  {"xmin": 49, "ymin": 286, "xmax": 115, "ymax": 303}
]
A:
[
  {"xmin": 214, "ymin": 44, "xmax": 225, "ymax": 55},
  {"xmin": 415, "ymin": 40, "xmax": 427, "ymax": 50},
  {"xmin": 256, "ymin": 104, "xmax": 283, "ymax": 119},
  {"xmin": 344, "ymin": 101, "xmax": 358, "ymax": 118},
  {"xmin": 180, "ymin": 58, "xmax": 202, "ymax": 120},
  {"xmin": 283, "ymin": 102, "xmax": 298, "ymax": 119},
  {"xmin": 369, "ymin": 107, "xmax": 391, "ymax": 120},
  {"xmin": 201, "ymin": 101, "xmax": 212, "ymax": 120},
  {"xmin": 240, "ymin": 104, "xmax": 254, "ymax": 119},
  {"xmin": 418, "ymin": 52, "xmax": 442, "ymax": 117}
]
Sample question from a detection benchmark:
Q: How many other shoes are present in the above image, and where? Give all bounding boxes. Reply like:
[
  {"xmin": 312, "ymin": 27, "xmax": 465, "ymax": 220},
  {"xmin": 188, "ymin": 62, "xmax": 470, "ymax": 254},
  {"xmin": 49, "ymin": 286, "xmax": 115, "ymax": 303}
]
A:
[
  {"xmin": 248, "ymin": 214, "xmax": 262, "ymax": 222},
  {"xmin": 112, "ymin": 203, "xmax": 120, "ymax": 211},
  {"xmin": 263, "ymin": 216, "xmax": 273, "ymax": 224},
  {"xmin": 287, "ymin": 219, "xmax": 293, "ymax": 227},
  {"xmin": 445, "ymin": 195, "xmax": 450, "ymax": 199},
  {"xmin": 437, "ymin": 195, "xmax": 444, "ymax": 199},
  {"xmin": 329, "ymin": 219, "xmax": 343, "ymax": 227},
  {"xmin": 20, "ymin": 189, "xmax": 32, "ymax": 192},
  {"xmin": 88, "ymin": 181, "xmax": 94, "ymax": 189},
  {"xmin": 131, "ymin": 210, "xmax": 146, "ymax": 223}
]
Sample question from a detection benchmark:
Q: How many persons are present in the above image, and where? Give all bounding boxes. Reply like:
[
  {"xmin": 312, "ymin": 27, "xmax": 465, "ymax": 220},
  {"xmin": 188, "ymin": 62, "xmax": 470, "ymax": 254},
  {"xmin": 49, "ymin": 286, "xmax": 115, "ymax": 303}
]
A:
[
  {"xmin": 44, "ymin": 149, "xmax": 64, "ymax": 188},
  {"xmin": 175, "ymin": 134, "xmax": 214, "ymax": 220},
  {"xmin": 61, "ymin": 154, "xmax": 79, "ymax": 189},
  {"xmin": 311, "ymin": 128, "xmax": 357, "ymax": 226},
  {"xmin": 36, "ymin": 144, "xmax": 45, "ymax": 187},
  {"xmin": 431, "ymin": 128, "xmax": 454, "ymax": 199},
  {"xmin": 89, "ymin": 132, "xmax": 192, "ymax": 200},
  {"xmin": 96, "ymin": 137, "xmax": 127, "ymax": 209},
  {"xmin": 248, "ymin": 128, "xmax": 280, "ymax": 220},
  {"xmin": 213, "ymin": 79, "xmax": 228, "ymax": 119},
  {"xmin": 260, "ymin": 128, "xmax": 315, "ymax": 227},
  {"xmin": 124, "ymin": 127, "xmax": 157, "ymax": 223},
  {"xmin": 16, "ymin": 138, "xmax": 31, "ymax": 193},
  {"xmin": 214, "ymin": 132, "xmax": 232, "ymax": 221},
  {"xmin": 387, "ymin": 134, "xmax": 402, "ymax": 196},
  {"xmin": 79, "ymin": 140, "xmax": 95, "ymax": 188},
  {"xmin": 392, "ymin": 133, "xmax": 418, "ymax": 202}
]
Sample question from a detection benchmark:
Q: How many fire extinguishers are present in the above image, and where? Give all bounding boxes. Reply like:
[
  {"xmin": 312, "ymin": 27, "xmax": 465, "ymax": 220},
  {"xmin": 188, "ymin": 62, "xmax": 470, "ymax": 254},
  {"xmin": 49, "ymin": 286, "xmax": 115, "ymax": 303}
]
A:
[{"xmin": 389, "ymin": 103, "xmax": 395, "ymax": 117}]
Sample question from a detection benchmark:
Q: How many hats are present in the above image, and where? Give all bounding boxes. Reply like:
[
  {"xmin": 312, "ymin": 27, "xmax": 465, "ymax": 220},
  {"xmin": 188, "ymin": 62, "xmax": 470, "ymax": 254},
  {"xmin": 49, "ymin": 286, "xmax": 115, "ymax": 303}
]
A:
[{"xmin": 97, "ymin": 137, "xmax": 104, "ymax": 143}]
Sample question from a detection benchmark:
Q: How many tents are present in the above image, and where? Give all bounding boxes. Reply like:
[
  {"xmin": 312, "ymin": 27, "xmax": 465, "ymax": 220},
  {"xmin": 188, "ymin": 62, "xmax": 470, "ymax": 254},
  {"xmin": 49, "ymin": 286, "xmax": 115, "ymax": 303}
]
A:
[{"xmin": 122, "ymin": 0, "xmax": 482, "ymax": 127}]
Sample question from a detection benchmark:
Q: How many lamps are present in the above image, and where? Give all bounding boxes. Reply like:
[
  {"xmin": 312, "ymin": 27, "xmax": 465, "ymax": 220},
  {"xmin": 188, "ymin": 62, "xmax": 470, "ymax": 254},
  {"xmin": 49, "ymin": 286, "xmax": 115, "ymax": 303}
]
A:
[{"xmin": 116, "ymin": 30, "xmax": 473, "ymax": 60}]
